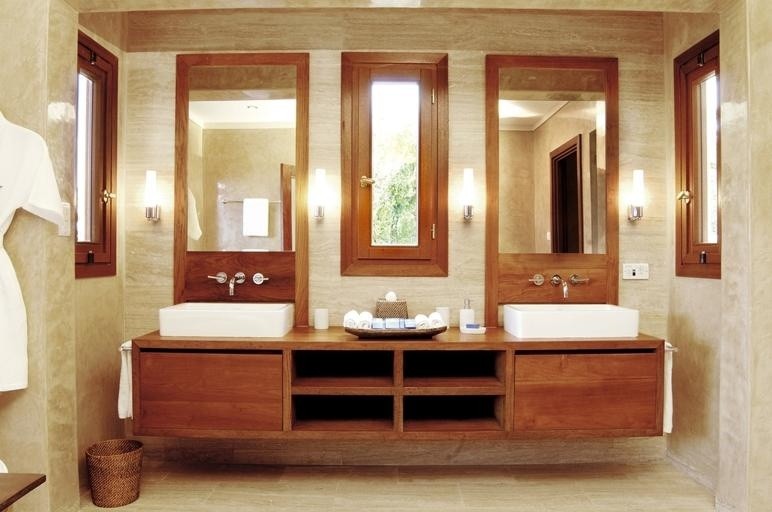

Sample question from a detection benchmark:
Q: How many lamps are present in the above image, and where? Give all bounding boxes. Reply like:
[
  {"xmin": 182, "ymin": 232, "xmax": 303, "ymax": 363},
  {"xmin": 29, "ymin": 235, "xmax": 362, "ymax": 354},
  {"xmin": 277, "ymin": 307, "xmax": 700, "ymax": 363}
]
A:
[
  {"xmin": 629, "ymin": 170, "xmax": 645, "ymax": 223},
  {"xmin": 144, "ymin": 170, "xmax": 158, "ymax": 221},
  {"xmin": 464, "ymin": 168, "xmax": 475, "ymax": 221},
  {"xmin": 314, "ymin": 169, "xmax": 326, "ymax": 219}
]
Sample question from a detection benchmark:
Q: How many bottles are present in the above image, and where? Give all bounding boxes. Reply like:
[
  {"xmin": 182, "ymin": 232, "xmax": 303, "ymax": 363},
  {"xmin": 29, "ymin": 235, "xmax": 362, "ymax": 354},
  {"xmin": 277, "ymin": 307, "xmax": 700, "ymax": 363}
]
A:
[{"xmin": 459, "ymin": 298, "xmax": 475, "ymax": 334}]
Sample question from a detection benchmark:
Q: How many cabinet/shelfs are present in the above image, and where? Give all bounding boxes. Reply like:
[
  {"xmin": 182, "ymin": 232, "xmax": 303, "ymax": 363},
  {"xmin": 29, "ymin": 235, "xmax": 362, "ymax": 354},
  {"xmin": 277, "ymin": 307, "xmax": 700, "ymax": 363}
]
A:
[
  {"xmin": 284, "ymin": 338, "xmax": 510, "ymax": 437},
  {"xmin": 133, "ymin": 338, "xmax": 283, "ymax": 437},
  {"xmin": 511, "ymin": 331, "xmax": 664, "ymax": 437}
]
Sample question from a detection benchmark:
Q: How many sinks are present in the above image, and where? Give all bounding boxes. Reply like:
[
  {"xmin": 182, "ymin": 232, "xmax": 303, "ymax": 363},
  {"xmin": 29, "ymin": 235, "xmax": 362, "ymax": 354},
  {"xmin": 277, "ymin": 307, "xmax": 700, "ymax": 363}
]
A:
[
  {"xmin": 502, "ymin": 304, "xmax": 640, "ymax": 339},
  {"xmin": 158, "ymin": 302, "xmax": 295, "ymax": 338}
]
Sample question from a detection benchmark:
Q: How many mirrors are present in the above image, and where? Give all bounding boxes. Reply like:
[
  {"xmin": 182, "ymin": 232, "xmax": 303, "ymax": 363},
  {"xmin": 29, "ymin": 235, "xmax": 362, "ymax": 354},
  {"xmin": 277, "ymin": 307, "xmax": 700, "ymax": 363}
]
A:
[
  {"xmin": 485, "ymin": 54, "xmax": 619, "ymax": 329},
  {"xmin": 176, "ymin": 53, "xmax": 309, "ymax": 330}
]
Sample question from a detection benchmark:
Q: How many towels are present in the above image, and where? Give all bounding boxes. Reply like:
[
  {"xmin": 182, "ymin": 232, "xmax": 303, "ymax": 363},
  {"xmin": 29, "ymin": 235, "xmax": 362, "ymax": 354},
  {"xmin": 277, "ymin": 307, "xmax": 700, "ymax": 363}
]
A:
[
  {"xmin": 664, "ymin": 341, "xmax": 673, "ymax": 433},
  {"xmin": 344, "ymin": 310, "xmax": 443, "ymax": 331},
  {"xmin": 117, "ymin": 340, "xmax": 133, "ymax": 419},
  {"xmin": 243, "ymin": 197, "xmax": 268, "ymax": 237}
]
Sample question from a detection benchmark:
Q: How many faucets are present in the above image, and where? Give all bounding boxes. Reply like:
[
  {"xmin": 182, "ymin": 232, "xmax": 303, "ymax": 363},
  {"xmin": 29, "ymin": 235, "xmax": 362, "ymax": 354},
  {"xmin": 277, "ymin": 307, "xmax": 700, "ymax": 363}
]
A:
[
  {"xmin": 228, "ymin": 272, "xmax": 246, "ymax": 296},
  {"xmin": 550, "ymin": 274, "xmax": 569, "ymax": 299}
]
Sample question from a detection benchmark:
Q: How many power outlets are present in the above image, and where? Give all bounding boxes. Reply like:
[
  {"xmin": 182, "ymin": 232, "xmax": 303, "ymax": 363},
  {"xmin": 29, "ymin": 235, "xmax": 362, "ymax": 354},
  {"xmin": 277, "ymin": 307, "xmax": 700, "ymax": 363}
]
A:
[{"xmin": 623, "ymin": 263, "xmax": 650, "ymax": 280}]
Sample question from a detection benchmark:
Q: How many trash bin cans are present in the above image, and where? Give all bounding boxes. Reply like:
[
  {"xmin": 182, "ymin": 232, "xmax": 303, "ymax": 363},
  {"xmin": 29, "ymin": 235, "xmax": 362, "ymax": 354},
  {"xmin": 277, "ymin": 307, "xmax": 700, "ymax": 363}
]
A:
[{"xmin": 85, "ymin": 439, "xmax": 144, "ymax": 508}]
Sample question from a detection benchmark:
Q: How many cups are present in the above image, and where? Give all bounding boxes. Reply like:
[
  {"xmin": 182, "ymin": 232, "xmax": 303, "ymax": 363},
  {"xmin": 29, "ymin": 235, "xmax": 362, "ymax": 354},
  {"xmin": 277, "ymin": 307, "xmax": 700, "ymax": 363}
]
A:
[
  {"xmin": 314, "ymin": 309, "xmax": 329, "ymax": 330},
  {"xmin": 436, "ymin": 307, "xmax": 450, "ymax": 329}
]
